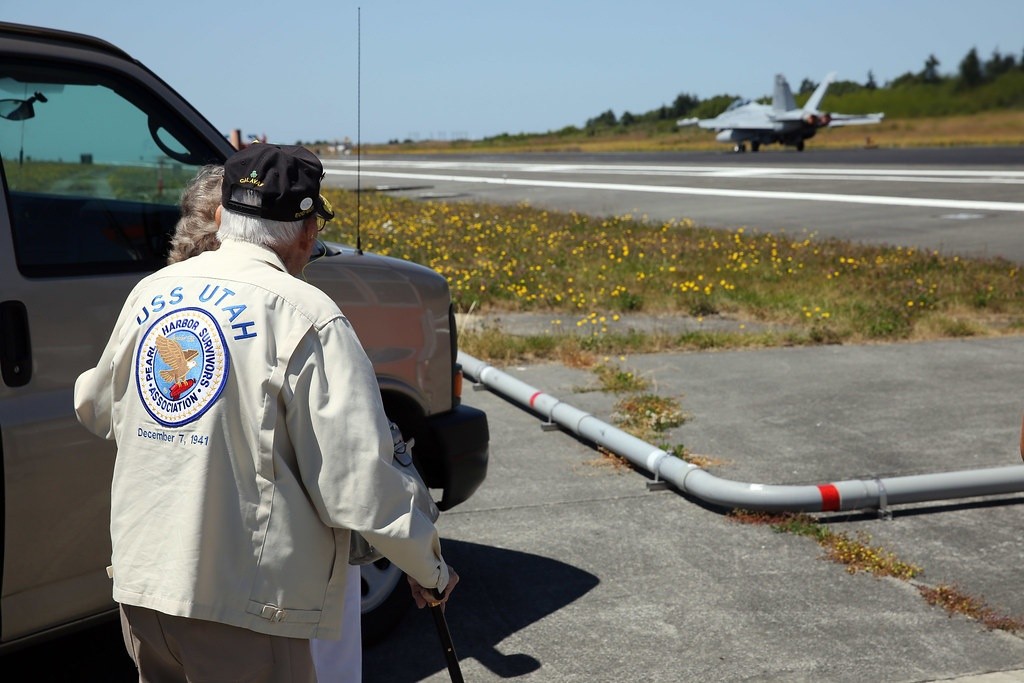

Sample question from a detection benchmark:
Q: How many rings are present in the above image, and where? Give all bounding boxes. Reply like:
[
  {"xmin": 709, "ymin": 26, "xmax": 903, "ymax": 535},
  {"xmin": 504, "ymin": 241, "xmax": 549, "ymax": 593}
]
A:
[{"xmin": 431, "ymin": 602, "xmax": 440, "ymax": 606}]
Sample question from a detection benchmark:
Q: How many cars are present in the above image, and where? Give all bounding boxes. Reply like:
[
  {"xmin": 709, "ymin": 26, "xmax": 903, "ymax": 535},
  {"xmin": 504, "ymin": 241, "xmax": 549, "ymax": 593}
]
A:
[{"xmin": 0, "ymin": 30, "xmax": 488, "ymax": 643}]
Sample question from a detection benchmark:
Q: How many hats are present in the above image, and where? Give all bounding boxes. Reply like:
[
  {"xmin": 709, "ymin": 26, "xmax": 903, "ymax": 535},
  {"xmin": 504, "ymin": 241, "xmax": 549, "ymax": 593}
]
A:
[{"xmin": 220, "ymin": 141, "xmax": 336, "ymax": 223}]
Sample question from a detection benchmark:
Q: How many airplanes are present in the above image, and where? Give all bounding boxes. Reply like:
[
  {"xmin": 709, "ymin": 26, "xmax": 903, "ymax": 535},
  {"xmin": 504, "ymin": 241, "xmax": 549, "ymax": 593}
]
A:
[{"xmin": 698, "ymin": 74, "xmax": 885, "ymax": 152}]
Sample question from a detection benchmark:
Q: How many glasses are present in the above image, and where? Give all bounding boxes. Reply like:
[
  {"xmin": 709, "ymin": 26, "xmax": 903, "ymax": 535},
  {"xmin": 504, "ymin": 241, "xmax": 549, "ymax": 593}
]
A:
[{"xmin": 315, "ymin": 213, "xmax": 326, "ymax": 230}]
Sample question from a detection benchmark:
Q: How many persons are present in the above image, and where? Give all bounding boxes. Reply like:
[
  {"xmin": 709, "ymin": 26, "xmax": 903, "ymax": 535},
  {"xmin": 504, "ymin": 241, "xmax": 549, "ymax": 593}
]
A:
[
  {"xmin": 169, "ymin": 165, "xmax": 361, "ymax": 683},
  {"xmin": 73, "ymin": 142, "xmax": 459, "ymax": 683}
]
